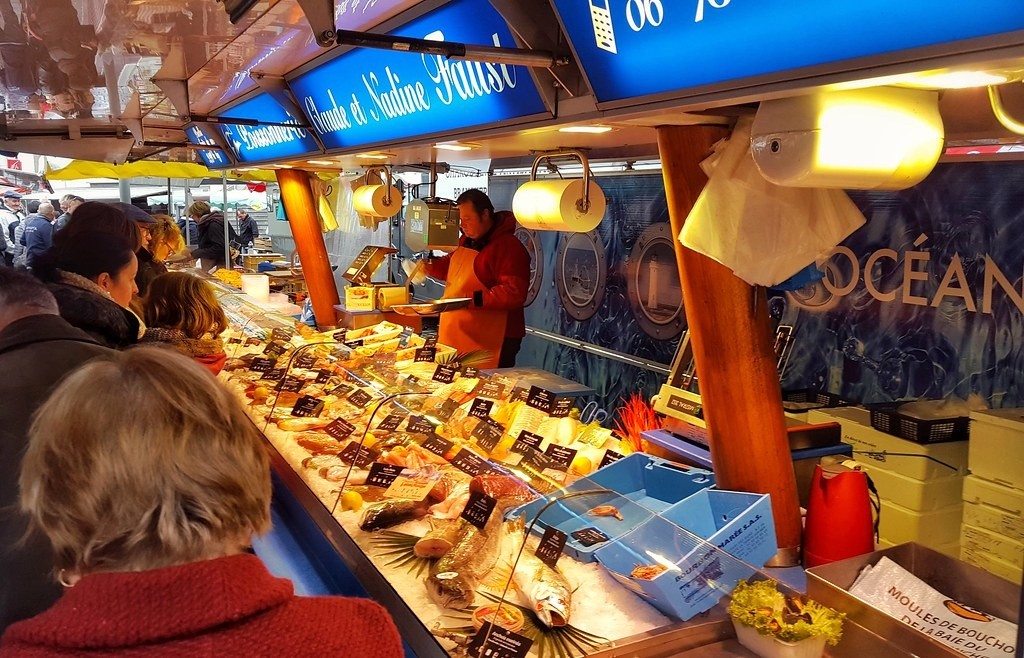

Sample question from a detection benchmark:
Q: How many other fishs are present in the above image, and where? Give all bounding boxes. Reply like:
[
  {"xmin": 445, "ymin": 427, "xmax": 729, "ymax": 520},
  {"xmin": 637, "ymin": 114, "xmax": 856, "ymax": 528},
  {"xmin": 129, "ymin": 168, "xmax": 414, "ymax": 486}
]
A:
[{"xmin": 223, "ymin": 330, "xmax": 572, "ymax": 626}]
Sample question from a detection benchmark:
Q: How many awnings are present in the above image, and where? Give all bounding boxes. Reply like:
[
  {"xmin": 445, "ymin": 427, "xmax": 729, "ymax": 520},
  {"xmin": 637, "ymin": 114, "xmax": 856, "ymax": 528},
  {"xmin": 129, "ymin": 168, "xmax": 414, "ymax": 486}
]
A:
[{"xmin": 45, "ymin": 155, "xmax": 284, "ymax": 182}]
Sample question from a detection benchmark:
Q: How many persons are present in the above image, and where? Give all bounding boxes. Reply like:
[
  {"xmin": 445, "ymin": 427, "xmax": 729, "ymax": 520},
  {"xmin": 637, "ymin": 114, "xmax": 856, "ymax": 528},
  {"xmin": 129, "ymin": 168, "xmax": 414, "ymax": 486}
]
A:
[
  {"xmin": 0, "ymin": 346, "xmax": 406, "ymax": 657},
  {"xmin": 1, "ymin": 189, "xmax": 261, "ymax": 521},
  {"xmin": 403, "ymin": 189, "xmax": 531, "ymax": 371},
  {"xmin": 0, "ymin": 1, "xmax": 116, "ymax": 120}
]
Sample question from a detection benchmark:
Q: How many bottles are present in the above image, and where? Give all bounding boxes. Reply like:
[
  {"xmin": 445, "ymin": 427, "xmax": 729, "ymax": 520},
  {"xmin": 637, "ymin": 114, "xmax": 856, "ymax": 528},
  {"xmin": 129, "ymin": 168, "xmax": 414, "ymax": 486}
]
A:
[{"xmin": 248, "ymin": 241, "xmax": 253, "ymax": 254}]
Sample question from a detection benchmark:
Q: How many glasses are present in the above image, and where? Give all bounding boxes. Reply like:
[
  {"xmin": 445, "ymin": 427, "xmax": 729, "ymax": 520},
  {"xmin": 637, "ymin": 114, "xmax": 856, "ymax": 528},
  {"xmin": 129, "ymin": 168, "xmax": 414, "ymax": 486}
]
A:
[
  {"xmin": 164, "ymin": 241, "xmax": 176, "ymax": 257},
  {"xmin": 60, "ymin": 207, "xmax": 67, "ymax": 211},
  {"xmin": 189, "ymin": 214, "xmax": 194, "ymax": 218},
  {"xmin": 139, "ymin": 226, "xmax": 151, "ymax": 236}
]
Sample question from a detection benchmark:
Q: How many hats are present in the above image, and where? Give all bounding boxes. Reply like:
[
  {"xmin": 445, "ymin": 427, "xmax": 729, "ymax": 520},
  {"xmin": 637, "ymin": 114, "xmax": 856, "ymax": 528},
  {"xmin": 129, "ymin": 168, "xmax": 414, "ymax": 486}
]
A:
[
  {"xmin": 4, "ymin": 191, "xmax": 22, "ymax": 198},
  {"xmin": 108, "ymin": 202, "xmax": 158, "ymax": 224}
]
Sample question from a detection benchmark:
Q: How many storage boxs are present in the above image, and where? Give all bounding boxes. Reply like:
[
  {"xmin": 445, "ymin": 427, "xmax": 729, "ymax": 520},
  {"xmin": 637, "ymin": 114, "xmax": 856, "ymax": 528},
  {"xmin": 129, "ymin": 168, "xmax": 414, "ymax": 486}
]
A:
[
  {"xmin": 344, "ymin": 285, "xmax": 375, "ymax": 312},
  {"xmin": 503, "ymin": 403, "xmax": 1024, "ymax": 658}
]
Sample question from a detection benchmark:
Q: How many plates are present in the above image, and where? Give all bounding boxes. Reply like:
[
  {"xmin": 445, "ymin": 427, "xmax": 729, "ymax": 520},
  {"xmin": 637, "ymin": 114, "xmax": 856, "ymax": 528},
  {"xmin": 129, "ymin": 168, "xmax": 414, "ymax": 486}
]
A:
[{"xmin": 390, "ymin": 298, "xmax": 472, "ymax": 316}]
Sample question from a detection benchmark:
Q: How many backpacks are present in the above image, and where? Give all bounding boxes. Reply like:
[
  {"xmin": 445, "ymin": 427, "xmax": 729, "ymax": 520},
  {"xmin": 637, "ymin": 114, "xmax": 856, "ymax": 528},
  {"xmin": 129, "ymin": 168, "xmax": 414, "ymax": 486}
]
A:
[{"xmin": 0, "ymin": 22, "xmax": 28, "ymax": 68}]
